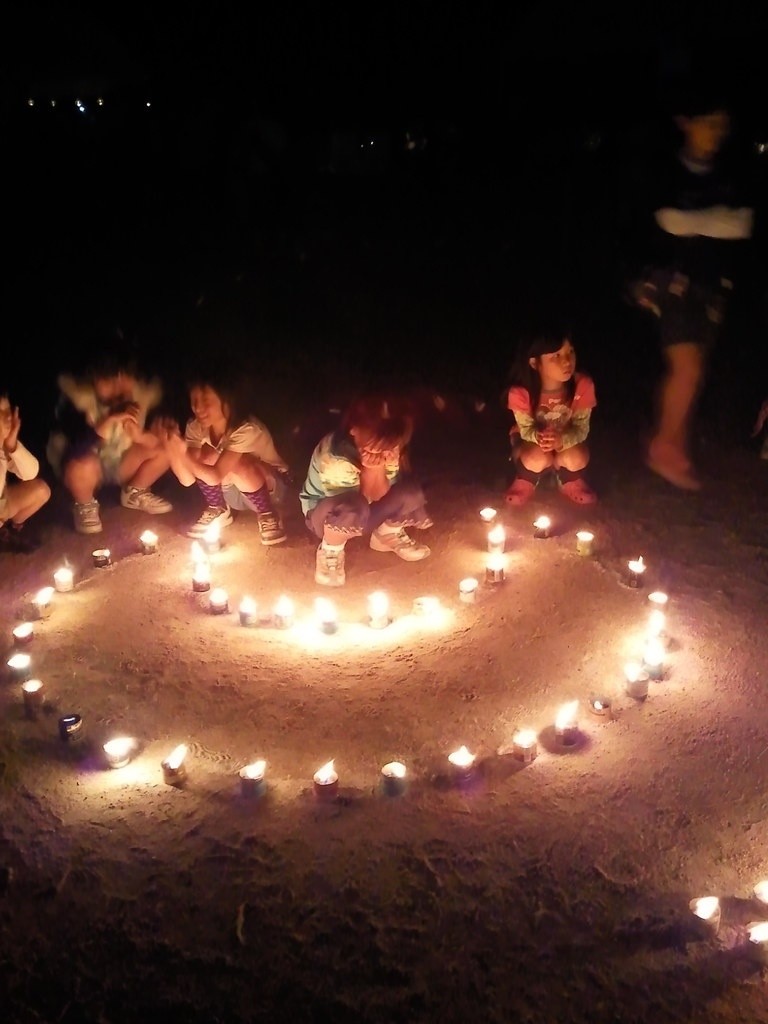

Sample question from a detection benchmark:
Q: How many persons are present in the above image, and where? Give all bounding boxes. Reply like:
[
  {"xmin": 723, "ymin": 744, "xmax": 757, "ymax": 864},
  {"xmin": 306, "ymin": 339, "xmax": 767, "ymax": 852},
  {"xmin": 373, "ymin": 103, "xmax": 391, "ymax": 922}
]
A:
[
  {"xmin": 44, "ymin": 346, "xmax": 173, "ymax": 533},
  {"xmin": 504, "ymin": 333, "xmax": 599, "ymax": 506},
  {"xmin": 628, "ymin": 95, "xmax": 760, "ymax": 488},
  {"xmin": 0, "ymin": 394, "xmax": 51, "ymax": 552},
  {"xmin": 153, "ymin": 371, "xmax": 294, "ymax": 546},
  {"xmin": 298, "ymin": 394, "xmax": 434, "ymax": 586}
]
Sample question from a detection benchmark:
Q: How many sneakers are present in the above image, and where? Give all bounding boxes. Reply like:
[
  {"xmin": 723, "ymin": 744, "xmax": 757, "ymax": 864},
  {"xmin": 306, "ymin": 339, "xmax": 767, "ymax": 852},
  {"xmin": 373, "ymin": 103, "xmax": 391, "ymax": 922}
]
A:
[
  {"xmin": 122, "ymin": 485, "xmax": 173, "ymax": 515},
  {"xmin": 315, "ymin": 542, "xmax": 346, "ymax": 587},
  {"xmin": 369, "ymin": 528, "xmax": 430, "ymax": 561},
  {"xmin": 257, "ymin": 510, "xmax": 286, "ymax": 545},
  {"xmin": 187, "ymin": 504, "xmax": 233, "ymax": 538},
  {"xmin": 72, "ymin": 498, "xmax": 102, "ymax": 534}
]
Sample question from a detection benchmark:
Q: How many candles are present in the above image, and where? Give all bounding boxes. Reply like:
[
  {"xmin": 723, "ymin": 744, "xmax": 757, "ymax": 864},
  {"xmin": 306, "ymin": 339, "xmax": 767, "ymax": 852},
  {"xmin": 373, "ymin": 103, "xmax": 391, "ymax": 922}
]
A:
[{"xmin": 5, "ymin": 506, "xmax": 677, "ymax": 798}]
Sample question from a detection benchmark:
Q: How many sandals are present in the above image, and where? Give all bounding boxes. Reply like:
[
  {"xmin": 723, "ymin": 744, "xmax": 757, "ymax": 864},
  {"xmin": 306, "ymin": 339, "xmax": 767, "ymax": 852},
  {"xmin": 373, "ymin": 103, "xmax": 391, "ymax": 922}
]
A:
[
  {"xmin": 556, "ymin": 476, "xmax": 596, "ymax": 505},
  {"xmin": 504, "ymin": 475, "xmax": 540, "ymax": 508}
]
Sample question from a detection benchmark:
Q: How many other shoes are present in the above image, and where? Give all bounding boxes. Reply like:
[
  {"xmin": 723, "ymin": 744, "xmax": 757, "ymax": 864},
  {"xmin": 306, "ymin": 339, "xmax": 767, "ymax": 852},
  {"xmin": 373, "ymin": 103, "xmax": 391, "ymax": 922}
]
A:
[
  {"xmin": 647, "ymin": 441, "xmax": 702, "ymax": 491},
  {"xmin": 9, "ymin": 524, "xmax": 38, "ymax": 551}
]
